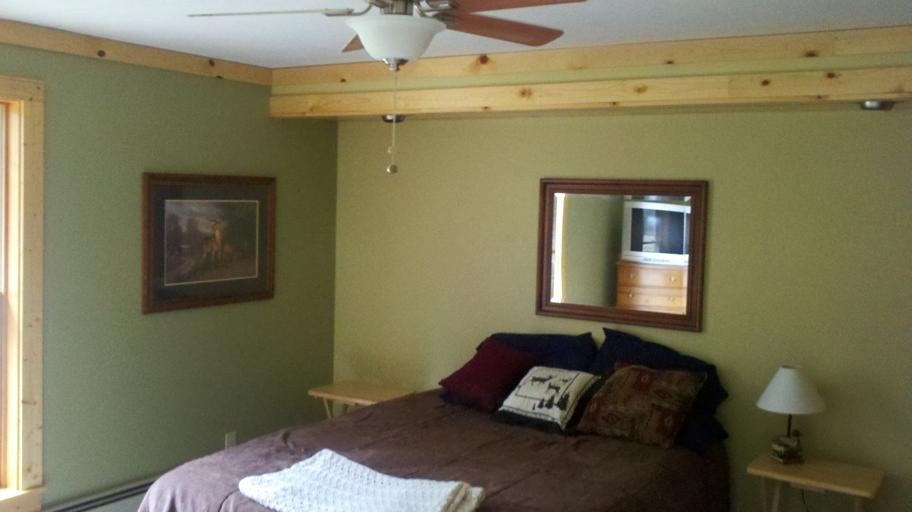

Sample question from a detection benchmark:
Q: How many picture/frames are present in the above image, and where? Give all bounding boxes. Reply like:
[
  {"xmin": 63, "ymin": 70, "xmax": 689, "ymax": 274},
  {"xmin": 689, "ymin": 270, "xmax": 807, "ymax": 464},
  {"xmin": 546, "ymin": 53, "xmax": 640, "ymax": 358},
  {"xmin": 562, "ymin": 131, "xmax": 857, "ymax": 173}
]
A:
[{"xmin": 141, "ymin": 169, "xmax": 277, "ymax": 314}]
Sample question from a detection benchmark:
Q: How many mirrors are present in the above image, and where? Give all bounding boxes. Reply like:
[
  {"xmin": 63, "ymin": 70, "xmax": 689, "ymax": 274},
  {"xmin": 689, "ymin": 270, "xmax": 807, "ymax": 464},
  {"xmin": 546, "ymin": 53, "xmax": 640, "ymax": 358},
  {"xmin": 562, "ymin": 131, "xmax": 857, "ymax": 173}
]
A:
[{"xmin": 535, "ymin": 175, "xmax": 710, "ymax": 333}]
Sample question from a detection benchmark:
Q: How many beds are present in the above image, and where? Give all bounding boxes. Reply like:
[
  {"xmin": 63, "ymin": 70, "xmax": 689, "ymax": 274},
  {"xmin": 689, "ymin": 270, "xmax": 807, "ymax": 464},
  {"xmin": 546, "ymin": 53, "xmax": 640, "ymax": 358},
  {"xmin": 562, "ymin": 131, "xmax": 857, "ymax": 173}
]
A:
[{"xmin": 143, "ymin": 385, "xmax": 733, "ymax": 512}]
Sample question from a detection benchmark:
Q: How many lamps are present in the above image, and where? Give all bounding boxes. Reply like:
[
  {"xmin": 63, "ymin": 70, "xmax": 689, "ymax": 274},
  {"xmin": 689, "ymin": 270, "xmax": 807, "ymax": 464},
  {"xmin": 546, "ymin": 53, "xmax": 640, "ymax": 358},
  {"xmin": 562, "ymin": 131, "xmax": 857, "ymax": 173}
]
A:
[
  {"xmin": 346, "ymin": 14, "xmax": 447, "ymax": 72},
  {"xmin": 756, "ymin": 365, "xmax": 827, "ymax": 464}
]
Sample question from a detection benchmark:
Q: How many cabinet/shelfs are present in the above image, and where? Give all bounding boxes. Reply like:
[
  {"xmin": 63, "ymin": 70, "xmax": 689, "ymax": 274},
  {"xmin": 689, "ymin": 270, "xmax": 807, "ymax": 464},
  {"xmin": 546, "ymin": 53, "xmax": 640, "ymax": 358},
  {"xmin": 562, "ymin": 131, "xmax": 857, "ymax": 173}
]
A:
[{"xmin": 612, "ymin": 258, "xmax": 688, "ymax": 315}]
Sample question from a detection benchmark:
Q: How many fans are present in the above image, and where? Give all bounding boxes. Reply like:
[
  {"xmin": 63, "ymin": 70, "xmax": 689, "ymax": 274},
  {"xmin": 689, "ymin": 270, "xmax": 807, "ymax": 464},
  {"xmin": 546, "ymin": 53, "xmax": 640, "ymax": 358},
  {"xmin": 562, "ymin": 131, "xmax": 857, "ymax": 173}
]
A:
[{"xmin": 184, "ymin": 1, "xmax": 589, "ymax": 74}]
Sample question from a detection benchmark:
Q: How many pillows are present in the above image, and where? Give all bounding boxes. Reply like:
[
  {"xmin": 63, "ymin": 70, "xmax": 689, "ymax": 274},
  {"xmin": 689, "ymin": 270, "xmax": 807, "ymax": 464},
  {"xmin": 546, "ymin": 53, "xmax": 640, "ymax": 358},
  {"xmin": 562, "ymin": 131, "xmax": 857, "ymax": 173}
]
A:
[{"xmin": 438, "ymin": 327, "xmax": 714, "ymax": 451}]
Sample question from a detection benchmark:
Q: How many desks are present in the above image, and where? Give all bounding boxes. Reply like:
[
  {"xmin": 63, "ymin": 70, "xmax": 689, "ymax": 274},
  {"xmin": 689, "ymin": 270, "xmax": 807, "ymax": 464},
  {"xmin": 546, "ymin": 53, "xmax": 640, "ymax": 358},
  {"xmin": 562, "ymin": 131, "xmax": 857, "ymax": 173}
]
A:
[
  {"xmin": 747, "ymin": 453, "xmax": 886, "ymax": 511},
  {"xmin": 308, "ymin": 384, "xmax": 416, "ymax": 420}
]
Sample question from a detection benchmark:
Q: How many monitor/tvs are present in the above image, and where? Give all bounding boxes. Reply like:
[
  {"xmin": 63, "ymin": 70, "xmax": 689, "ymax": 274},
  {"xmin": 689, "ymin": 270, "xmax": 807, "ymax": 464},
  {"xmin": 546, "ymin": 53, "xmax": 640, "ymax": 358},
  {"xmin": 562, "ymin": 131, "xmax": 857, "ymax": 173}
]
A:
[{"xmin": 620, "ymin": 200, "xmax": 691, "ymax": 267}]
